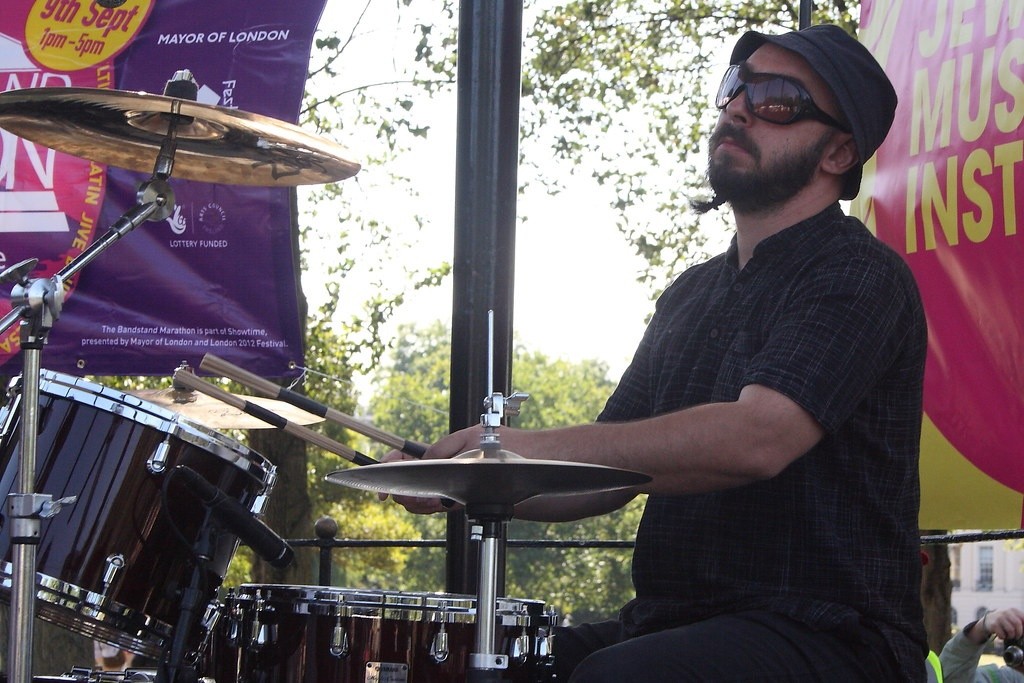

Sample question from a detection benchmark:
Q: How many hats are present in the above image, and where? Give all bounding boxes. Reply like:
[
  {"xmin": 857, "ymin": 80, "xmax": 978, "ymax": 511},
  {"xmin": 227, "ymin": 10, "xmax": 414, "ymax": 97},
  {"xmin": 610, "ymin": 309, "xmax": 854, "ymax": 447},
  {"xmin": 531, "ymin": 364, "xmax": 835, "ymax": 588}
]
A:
[{"xmin": 729, "ymin": 23, "xmax": 897, "ymax": 202}]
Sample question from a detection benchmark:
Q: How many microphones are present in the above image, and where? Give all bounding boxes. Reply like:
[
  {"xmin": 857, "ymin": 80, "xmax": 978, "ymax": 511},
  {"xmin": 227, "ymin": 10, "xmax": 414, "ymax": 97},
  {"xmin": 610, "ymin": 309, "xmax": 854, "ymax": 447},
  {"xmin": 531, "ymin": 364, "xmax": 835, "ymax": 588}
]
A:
[{"xmin": 173, "ymin": 464, "xmax": 295, "ymax": 571}]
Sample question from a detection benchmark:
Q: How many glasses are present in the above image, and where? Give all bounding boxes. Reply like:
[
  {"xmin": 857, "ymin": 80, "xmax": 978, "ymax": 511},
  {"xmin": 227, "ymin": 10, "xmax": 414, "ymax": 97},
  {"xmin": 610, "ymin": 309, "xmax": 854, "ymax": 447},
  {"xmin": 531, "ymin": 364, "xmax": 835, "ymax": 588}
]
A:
[{"xmin": 715, "ymin": 64, "xmax": 849, "ymax": 133}]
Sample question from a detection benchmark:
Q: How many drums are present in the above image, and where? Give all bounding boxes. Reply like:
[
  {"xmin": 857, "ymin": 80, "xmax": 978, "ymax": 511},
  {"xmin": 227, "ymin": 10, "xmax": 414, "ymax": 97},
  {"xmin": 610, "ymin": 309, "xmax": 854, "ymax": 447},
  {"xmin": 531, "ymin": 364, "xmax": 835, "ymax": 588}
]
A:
[
  {"xmin": 0, "ymin": 367, "xmax": 276, "ymax": 659},
  {"xmin": 211, "ymin": 583, "xmax": 559, "ymax": 683}
]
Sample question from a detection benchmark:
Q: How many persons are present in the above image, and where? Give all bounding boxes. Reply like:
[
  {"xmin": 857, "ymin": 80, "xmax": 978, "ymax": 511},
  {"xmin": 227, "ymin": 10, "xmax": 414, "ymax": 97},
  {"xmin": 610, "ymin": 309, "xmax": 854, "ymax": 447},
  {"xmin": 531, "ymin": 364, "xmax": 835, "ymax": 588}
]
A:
[
  {"xmin": 921, "ymin": 549, "xmax": 1024, "ymax": 683},
  {"xmin": 379, "ymin": 24, "xmax": 931, "ymax": 683}
]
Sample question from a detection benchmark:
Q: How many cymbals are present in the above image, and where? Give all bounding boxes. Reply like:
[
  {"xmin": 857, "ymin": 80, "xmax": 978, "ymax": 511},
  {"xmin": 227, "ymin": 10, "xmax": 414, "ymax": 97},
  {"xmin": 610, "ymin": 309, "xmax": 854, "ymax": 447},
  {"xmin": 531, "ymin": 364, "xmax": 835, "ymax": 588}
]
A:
[
  {"xmin": 325, "ymin": 446, "xmax": 650, "ymax": 508},
  {"xmin": 118, "ymin": 387, "xmax": 328, "ymax": 430},
  {"xmin": 0, "ymin": 87, "xmax": 361, "ymax": 186}
]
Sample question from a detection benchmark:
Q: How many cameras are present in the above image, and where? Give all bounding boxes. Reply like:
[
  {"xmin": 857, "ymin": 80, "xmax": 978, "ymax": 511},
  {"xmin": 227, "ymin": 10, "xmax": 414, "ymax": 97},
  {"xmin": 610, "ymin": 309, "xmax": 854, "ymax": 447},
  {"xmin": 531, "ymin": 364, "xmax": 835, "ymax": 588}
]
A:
[{"xmin": 1002, "ymin": 634, "xmax": 1024, "ymax": 674}]
